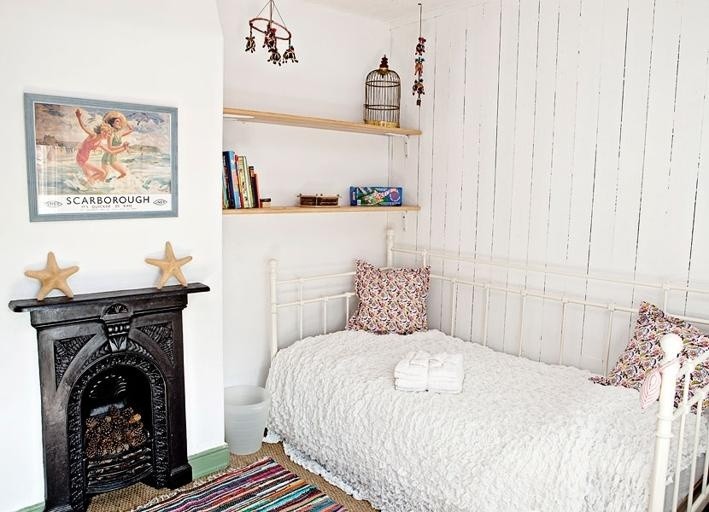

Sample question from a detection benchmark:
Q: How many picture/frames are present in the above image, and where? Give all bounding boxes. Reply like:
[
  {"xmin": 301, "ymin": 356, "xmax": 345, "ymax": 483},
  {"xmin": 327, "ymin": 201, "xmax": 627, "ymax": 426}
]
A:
[{"xmin": 23, "ymin": 94, "xmax": 179, "ymax": 221}]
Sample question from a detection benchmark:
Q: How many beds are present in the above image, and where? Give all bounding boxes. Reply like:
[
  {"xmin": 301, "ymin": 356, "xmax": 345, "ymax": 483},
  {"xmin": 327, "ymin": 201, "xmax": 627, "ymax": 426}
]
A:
[{"xmin": 266, "ymin": 225, "xmax": 708, "ymax": 512}]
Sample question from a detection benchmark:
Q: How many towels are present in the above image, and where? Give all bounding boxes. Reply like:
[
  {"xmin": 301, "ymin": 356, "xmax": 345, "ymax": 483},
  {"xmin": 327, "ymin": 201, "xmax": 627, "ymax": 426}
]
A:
[{"xmin": 393, "ymin": 348, "xmax": 467, "ymax": 393}]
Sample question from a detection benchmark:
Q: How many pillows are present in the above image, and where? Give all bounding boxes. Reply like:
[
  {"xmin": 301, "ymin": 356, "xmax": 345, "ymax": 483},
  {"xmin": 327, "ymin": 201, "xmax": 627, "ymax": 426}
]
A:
[
  {"xmin": 346, "ymin": 255, "xmax": 430, "ymax": 333},
  {"xmin": 590, "ymin": 298, "xmax": 708, "ymax": 417}
]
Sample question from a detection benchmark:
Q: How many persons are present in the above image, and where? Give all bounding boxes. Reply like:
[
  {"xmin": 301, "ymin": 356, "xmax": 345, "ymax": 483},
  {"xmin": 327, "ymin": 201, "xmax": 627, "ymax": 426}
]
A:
[
  {"xmin": 99, "ymin": 111, "xmax": 134, "ymax": 182},
  {"xmin": 71, "ymin": 108, "xmax": 130, "ymax": 192}
]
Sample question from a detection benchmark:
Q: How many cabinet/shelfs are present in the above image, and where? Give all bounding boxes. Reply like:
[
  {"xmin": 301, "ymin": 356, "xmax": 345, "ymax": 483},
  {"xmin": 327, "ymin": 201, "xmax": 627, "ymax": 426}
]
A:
[{"xmin": 222, "ymin": 107, "xmax": 422, "ymax": 214}]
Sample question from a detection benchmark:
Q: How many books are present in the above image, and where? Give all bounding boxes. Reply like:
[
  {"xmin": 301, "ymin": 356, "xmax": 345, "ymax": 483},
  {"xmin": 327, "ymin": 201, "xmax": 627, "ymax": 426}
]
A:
[{"xmin": 221, "ymin": 150, "xmax": 261, "ymax": 210}]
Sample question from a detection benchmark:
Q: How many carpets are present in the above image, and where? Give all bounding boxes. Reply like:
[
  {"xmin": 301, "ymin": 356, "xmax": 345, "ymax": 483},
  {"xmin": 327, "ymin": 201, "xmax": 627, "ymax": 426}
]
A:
[{"xmin": 122, "ymin": 455, "xmax": 352, "ymax": 512}]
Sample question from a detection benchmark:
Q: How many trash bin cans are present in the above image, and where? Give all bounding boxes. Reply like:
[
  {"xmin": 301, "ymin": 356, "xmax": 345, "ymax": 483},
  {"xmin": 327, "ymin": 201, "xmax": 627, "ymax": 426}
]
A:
[{"xmin": 225, "ymin": 385, "xmax": 271, "ymax": 456}]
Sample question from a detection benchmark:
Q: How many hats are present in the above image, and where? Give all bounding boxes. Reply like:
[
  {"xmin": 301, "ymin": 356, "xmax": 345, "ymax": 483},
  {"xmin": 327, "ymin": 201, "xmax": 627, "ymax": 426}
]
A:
[{"xmin": 103, "ymin": 110, "xmax": 128, "ymax": 130}]
